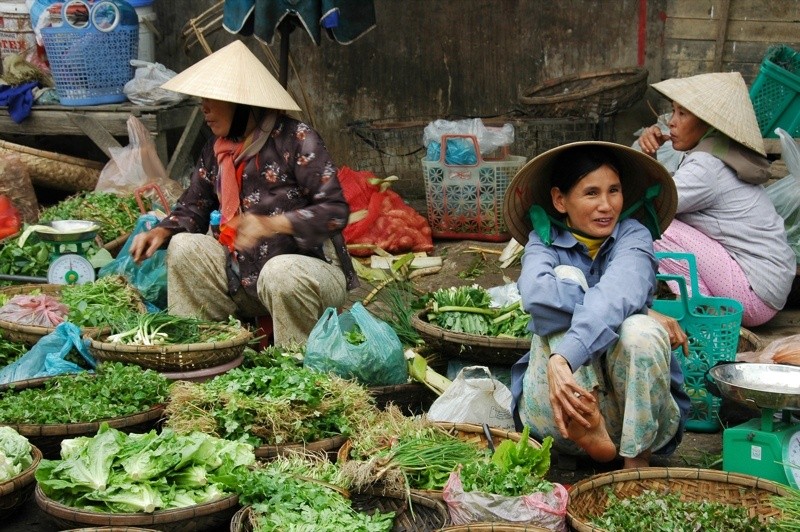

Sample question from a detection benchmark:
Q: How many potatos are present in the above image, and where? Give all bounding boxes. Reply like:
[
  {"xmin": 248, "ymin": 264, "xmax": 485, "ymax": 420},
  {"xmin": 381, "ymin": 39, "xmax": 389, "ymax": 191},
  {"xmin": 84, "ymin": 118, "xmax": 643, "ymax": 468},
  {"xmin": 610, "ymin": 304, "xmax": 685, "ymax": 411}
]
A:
[{"xmin": 347, "ymin": 198, "xmax": 434, "ymax": 254}]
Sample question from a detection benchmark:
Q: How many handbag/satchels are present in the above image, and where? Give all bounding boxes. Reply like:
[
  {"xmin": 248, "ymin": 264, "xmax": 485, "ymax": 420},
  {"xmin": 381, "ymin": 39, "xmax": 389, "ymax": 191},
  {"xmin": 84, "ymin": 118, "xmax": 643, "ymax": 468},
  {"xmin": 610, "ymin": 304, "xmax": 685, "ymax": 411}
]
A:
[
  {"xmin": 421, "ymin": 135, "xmax": 525, "ymax": 241},
  {"xmin": 430, "ymin": 365, "xmax": 515, "ymax": 433},
  {"xmin": 763, "ymin": 129, "xmax": 800, "ymax": 263},
  {"xmin": 98, "ymin": 217, "xmax": 168, "ymax": 299},
  {"xmin": 96, "ymin": 114, "xmax": 182, "ymax": 206},
  {"xmin": 443, "ymin": 464, "xmax": 568, "ymax": 532},
  {"xmin": 650, "ymin": 250, "xmax": 742, "ymax": 429},
  {"xmin": 304, "ymin": 303, "xmax": 407, "ymax": 385},
  {"xmin": 0, "ymin": 293, "xmax": 65, "ymax": 330},
  {"xmin": 0, "ymin": 322, "xmax": 97, "ymax": 384},
  {"xmin": 41, "ymin": 0, "xmax": 138, "ymax": 107}
]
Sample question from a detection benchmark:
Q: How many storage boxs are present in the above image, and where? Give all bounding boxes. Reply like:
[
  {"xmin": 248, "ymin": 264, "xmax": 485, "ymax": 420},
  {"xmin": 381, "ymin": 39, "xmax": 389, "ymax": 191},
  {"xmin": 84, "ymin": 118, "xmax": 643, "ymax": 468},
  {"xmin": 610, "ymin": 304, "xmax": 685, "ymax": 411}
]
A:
[{"xmin": 747, "ymin": 44, "xmax": 800, "ymax": 139}]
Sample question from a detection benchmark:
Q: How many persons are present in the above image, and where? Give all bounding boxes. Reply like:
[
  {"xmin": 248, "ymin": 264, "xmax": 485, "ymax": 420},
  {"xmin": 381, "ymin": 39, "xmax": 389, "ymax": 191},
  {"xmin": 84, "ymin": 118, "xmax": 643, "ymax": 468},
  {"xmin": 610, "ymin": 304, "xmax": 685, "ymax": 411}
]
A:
[
  {"xmin": 637, "ymin": 72, "xmax": 796, "ymax": 327},
  {"xmin": 502, "ymin": 141, "xmax": 691, "ymax": 469},
  {"xmin": 129, "ymin": 40, "xmax": 360, "ymax": 350}
]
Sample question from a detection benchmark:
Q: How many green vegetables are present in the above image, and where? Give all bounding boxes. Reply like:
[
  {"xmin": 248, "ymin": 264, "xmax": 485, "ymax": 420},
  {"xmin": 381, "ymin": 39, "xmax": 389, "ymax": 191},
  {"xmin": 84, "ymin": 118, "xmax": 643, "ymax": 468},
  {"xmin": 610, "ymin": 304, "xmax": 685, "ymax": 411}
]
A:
[
  {"xmin": 0, "ymin": 191, "xmax": 151, "ymax": 328},
  {"xmin": 427, "ymin": 286, "xmax": 531, "ymax": 337},
  {"xmin": 0, "ymin": 323, "xmax": 800, "ymax": 532}
]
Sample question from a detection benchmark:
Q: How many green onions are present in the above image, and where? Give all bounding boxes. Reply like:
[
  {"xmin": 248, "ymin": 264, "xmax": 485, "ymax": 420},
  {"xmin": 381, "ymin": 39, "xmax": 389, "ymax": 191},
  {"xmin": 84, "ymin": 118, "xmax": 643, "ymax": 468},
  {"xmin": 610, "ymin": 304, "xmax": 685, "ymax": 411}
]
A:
[
  {"xmin": 99, "ymin": 302, "xmax": 235, "ymax": 344},
  {"xmin": 351, "ymin": 258, "xmax": 430, "ymax": 349}
]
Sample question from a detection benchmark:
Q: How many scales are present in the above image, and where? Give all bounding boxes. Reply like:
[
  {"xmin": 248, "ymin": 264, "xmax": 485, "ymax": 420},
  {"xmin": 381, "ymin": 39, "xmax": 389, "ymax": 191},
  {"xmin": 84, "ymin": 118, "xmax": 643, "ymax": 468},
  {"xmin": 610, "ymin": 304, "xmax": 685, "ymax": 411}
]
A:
[
  {"xmin": 708, "ymin": 363, "xmax": 800, "ymax": 493},
  {"xmin": 35, "ymin": 220, "xmax": 100, "ymax": 284}
]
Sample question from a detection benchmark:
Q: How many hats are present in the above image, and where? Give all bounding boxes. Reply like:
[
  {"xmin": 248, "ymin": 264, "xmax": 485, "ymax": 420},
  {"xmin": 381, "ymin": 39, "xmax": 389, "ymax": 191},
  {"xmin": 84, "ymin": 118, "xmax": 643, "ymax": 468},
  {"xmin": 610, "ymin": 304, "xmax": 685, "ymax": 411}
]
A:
[
  {"xmin": 650, "ymin": 71, "xmax": 768, "ymax": 157},
  {"xmin": 159, "ymin": 40, "xmax": 302, "ymax": 114},
  {"xmin": 503, "ymin": 139, "xmax": 677, "ymax": 248}
]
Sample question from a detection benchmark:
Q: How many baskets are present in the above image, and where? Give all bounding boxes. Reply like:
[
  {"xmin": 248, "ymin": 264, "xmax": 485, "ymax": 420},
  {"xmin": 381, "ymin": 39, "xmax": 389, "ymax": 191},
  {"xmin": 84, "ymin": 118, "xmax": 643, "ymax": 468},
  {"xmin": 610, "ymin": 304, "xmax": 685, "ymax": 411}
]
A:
[
  {"xmin": 519, "ymin": 68, "xmax": 650, "ymax": 120},
  {"xmin": 749, "ymin": 45, "xmax": 800, "ymax": 138},
  {"xmin": 0, "ymin": 283, "xmax": 800, "ymax": 532}
]
